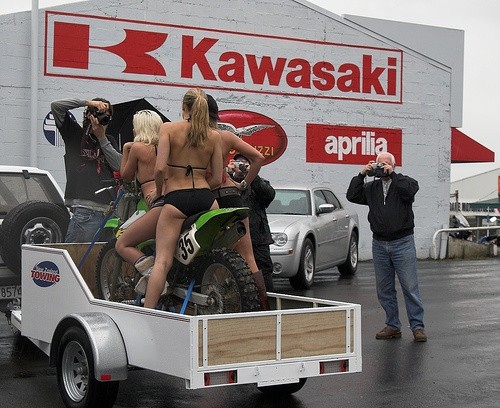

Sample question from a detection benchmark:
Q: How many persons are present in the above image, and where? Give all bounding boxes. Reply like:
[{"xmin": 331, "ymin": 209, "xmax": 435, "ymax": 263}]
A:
[
  {"xmin": 51, "ymin": 97, "xmax": 123, "ymax": 243},
  {"xmin": 204, "ymin": 93, "xmax": 270, "ymax": 311},
  {"xmin": 143, "ymin": 88, "xmax": 223, "ymax": 309},
  {"xmin": 225, "ymin": 153, "xmax": 277, "ymax": 311},
  {"xmin": 115, "ymin": 110, "xmax": 170, "ymax": 297},
  {"xmin": 345, "ymin": 151, "xmax": 428, "ymax": 342}
]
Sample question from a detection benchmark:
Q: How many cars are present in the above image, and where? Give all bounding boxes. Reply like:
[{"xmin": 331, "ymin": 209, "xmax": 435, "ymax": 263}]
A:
[
  {"xmin": 477, "ymin": 234, "xmax": 500, "ymax": 247},
  {"xmin": 263, "ymin": 183, "xmax": 361, "ymax": 290}
]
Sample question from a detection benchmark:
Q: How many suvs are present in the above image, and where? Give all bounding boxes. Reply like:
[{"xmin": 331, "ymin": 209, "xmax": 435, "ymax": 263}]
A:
[{"xmin": 449, "ymin": 211, "xmax": 472, "ymax": 240}]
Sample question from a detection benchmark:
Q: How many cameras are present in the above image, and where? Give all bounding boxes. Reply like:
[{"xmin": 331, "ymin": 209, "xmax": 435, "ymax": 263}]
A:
[
  {"xmin": 368, "ymin": 163, "xmax": 389, "ymax": 178},
  {"xmin": 232, "ymin": 160, "xmax": 246, "ymax": 171},
  {"xmin": 91, "ymin": 110, "xmax": 111, "ymax": 126}
]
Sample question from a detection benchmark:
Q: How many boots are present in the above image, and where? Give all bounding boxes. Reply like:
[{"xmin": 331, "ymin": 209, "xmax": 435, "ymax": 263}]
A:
[
  {"xmin": 251, "ymin": 270, "xmax": 270, "ymax": 311},
  {"xmin": 133, "ymin": 255, "xmax": 171, "ymax": 304}
]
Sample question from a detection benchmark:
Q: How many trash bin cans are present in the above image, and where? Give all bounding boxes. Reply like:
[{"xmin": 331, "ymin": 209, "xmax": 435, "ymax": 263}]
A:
[{"xmin": 489, "ymin": 242, "xmax": 497, "ymax": 257}]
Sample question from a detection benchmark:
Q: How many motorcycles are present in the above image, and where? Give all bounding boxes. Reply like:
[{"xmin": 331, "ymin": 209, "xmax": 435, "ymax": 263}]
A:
[{"xmin": 94, "ymin": 172, "xmax": 263, "ymax": 316}]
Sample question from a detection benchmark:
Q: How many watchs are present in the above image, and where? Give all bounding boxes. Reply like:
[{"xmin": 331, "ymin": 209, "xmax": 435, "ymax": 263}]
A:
[{"xmin": 241, "ymin": 179, "xmax": 251, "ymax": 190}]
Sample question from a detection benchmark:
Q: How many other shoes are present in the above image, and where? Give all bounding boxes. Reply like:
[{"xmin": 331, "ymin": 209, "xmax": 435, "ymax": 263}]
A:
[
  {"xmin": 375, "ymin": 325, "xmax": 402, "ymax": 340},
  {"xmin": 412, "ymin": 328, "xmax": 427, "ymax": 342}
]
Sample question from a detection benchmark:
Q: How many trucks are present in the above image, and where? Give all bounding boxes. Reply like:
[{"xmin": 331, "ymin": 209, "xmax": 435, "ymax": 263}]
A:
[{"xmin": 0, "ymin": 165, "xmax": 362, "ymax": 408}]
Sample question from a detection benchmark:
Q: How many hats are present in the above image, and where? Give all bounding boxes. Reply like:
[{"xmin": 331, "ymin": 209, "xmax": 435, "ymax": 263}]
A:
[
  {"xmin": 233, "ymin": 151, "xmax": 251, "ymax": 165},
  {"xmin": 206, "ymin": 95, "xmax": 219, "ymax": 118}
]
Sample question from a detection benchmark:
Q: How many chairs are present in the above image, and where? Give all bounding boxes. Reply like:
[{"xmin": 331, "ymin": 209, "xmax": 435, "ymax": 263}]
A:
[{"xmin": 268, "ymin": 197, "xmax": 305, "ymax": 213}]
